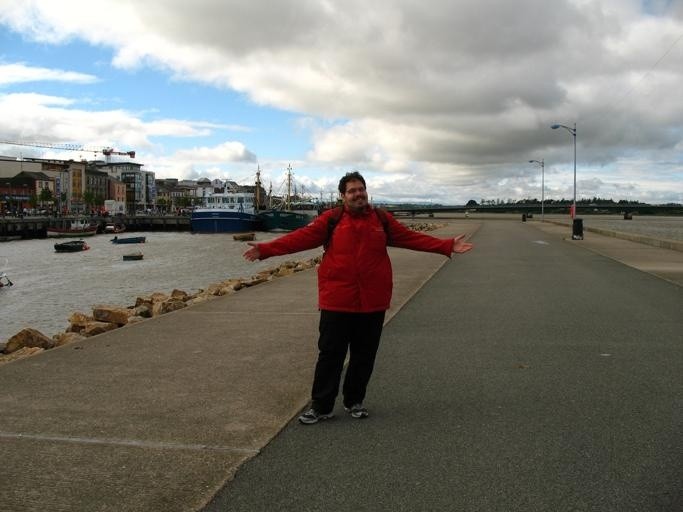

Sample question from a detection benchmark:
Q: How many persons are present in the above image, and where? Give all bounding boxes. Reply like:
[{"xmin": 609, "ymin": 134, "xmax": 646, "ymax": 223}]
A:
[{"xmin": 242, "ymin": 172, "xmax": 473, "ymax": 425}]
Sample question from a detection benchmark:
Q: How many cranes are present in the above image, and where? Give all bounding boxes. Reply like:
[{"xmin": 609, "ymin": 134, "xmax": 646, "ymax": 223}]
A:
[{"xmin": 0, "ymin": 138, "xmax": 135, "ymax": 164}]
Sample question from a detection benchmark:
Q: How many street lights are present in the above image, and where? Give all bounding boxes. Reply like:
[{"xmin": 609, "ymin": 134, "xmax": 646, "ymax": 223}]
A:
[
  {"xmin": 528, "ymin": 157, "xmax": 545, "ymax": 223},
  {"xmin": 548, "ymin": 121, "xmax": 576, "ymax": 218}
]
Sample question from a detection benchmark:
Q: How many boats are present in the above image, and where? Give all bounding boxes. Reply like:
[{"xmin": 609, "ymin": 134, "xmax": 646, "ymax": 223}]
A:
[
  {"xmin": 109, "ymin": 236, "xmax": 147, "ymax": 244},
  {"xmin": 54, "ymin": 238, "xmax": 90, "ymax": 252},
  {"xmin": 121, "ymin": 252, "xmax": 145, "ymax": 262},
  {"xmin": 45, "ymin": 211, "xmax": 99, "ymax": 237},
  {"xmin": 187, "ymin": 164, "xmax": 322, "ymax": 233},
  {"xmin": 101, "ymin": 222, "xmax": 126, "ymax": 233}
]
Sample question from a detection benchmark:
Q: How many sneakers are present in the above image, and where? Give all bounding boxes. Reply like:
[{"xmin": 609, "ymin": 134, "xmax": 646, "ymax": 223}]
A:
[
  {"xmin": 344, "ymin": 404, "xmax": 368, "ymax": 417},
  {"xmin": 297, "ymin": 406, "xmax": 335, "ymax": 423}
]
